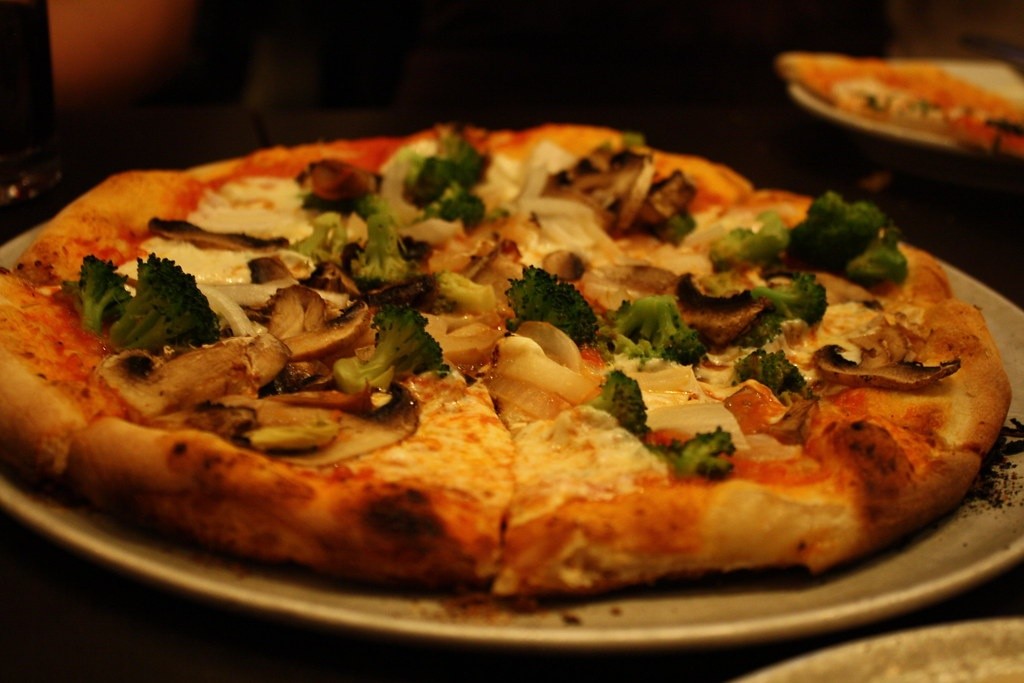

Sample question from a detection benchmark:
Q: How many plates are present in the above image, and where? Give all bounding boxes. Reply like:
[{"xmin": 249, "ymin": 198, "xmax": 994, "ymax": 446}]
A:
[
  {"xmin": 0, "ymin": 160, "xmax": 1024, "ymax": 650},
  {"xmin": 727, "ymin": 617, "xmax": 1024, "ymax": 683},
  {"xmin": 790, "ymin": 83, "xmax": 961, "ymax": 151}
]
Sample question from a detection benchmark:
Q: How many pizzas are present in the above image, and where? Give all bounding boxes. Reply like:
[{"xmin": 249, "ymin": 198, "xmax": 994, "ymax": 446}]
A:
[
  {"xmin": 0, "ymin": 121, "xmax": 1012, "ymax": 595},
  {"xmin": 777, "ymin": 51, "xmax": 1024, "ymax": 160}
]
